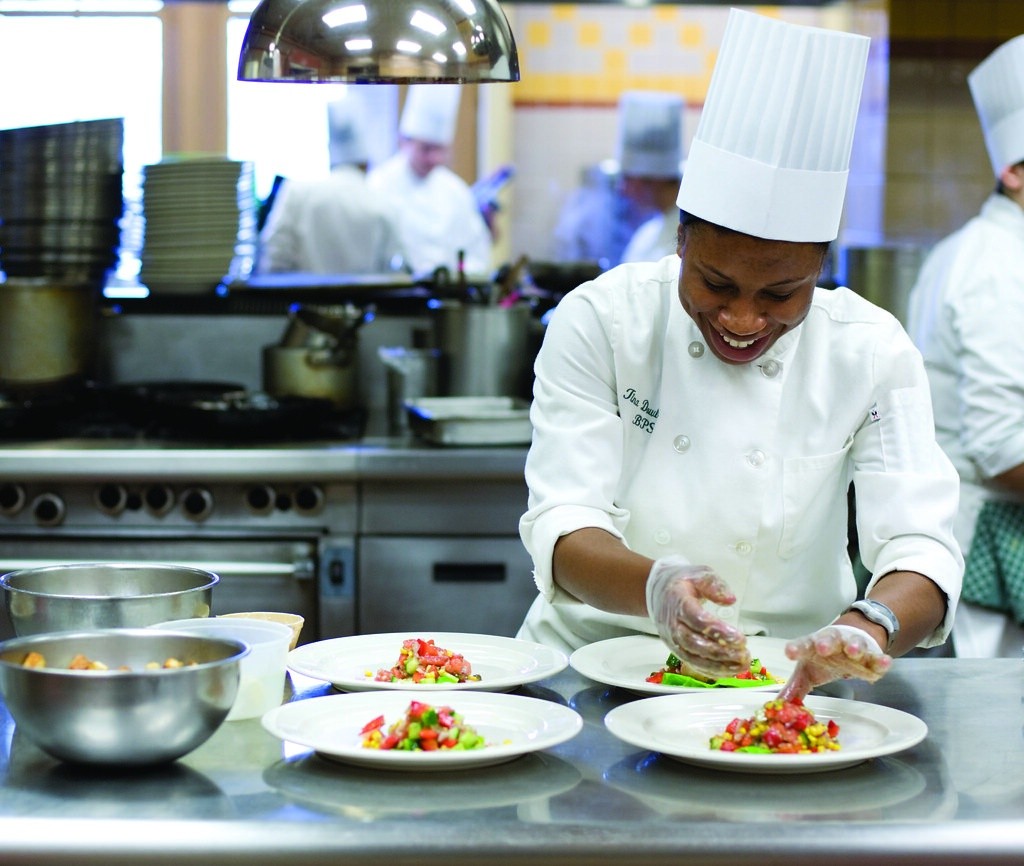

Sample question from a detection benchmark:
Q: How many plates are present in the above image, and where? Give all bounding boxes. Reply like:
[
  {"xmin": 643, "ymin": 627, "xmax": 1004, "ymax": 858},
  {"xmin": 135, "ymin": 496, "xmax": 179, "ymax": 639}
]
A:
[
  {"xmin": 260, "ymin": 690, "xmax": 583, "ymax": 770},
  {"xmin": 262, "ymin": 751, "xmax": 580, "ymax": 815},
  {"xmin": 604, "ymin": 690, "xmax": 929, "ymax": 776},
  {"xmin": 567, "ymin": 632, "xmax": 833, "ymax": 693},
  {"xmin": 284, "ymin": 632, "xmax": 569, "ymax": 694},
  {"xmin": 136, "ymin": 155, "xmax": 260, "ymax": 295},
  {"xmin": 605, "ymin": 747, "xmax": 927, "ymax": 814}
]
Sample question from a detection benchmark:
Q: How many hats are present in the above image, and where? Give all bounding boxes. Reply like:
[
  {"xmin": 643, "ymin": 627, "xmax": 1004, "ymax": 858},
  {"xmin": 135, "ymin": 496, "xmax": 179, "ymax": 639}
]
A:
[
  {"xmin": 620, "ymin": 92, "xmax": 684, "ymax": 179},
  {"xmin": 397, "ymin": 83, "xmax": 462, "ymax": 145},
  {"xmin": 967, "ymin": 34, "xmax": 1024, "ymax": 177},
  {"xmin": 328, "ymin": 106, "xmax": 370, "ymax": 167},
  {"xmin": 676, "ymin": 7, "xmax": 871, "ymax": 242}
]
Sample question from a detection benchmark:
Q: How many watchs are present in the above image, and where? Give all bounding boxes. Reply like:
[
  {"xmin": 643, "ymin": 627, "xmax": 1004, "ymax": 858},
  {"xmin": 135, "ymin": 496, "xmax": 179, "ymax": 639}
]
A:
[{"xmin": 838, "ymin": 600, "xmax": 900, "ymax": 656}]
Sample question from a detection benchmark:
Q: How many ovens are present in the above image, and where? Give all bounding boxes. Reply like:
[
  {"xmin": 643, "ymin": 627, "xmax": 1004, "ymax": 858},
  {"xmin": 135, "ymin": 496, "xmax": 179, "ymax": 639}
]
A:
[{"xmin": 0, "ymin": 526, "xmax": 358, "ymax": 643}]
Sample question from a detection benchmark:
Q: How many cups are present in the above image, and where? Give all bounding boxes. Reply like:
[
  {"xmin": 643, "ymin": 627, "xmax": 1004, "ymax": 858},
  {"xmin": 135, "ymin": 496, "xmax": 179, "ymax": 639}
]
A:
[{"xmin": 431, "ymin": 300, "xmax": 528, "ymax": 399}]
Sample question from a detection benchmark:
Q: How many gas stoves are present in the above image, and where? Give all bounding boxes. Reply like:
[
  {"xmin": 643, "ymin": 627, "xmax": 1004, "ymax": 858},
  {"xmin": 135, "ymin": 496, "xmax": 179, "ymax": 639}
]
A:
[{"xmin": 0, "ymin": 304, "xmax": 377, "ymax": 534}]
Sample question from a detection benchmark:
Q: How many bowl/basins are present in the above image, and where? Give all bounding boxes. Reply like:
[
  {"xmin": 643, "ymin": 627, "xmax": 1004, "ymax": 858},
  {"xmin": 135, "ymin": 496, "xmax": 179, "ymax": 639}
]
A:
[
  {"xmin": 148, "ymin": 616, "xmax": 292, "ymax": 721},
  {"xmin": 0, "ymin": 626, "xmax": 253, "ymax": 767},
  {"xmin": 0, "ymin": 116, "xmax": 125, "ymax": 289},
  {"xmin": 0, "ymin": 564, "xmax": 218, "ymax": 648},
  {"xmin": 223, "ymin": 611, "xmax": 305, "ymax": 650}
]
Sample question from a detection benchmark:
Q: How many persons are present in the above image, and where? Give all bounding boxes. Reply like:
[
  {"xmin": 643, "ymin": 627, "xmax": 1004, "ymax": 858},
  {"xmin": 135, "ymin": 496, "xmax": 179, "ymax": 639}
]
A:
[
  {"xmin": 515, "ymin": 5, "xmax": 964, "ymax": 708},
  {"xmin": 900, "ymin": 36, "xmax": 1024, "ymax": 660},
  {"xmin": 258, "ymin": 78, "xmax": 494, "ymax": 278},
  {"xmin": 618, "ymin": 89, "xmax": 685, "ymax": 267}
]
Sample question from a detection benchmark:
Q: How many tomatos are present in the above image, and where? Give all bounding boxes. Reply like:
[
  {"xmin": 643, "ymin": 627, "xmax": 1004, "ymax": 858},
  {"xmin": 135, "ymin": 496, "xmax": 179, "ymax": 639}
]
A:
[
  {"xmin": 377, "ymin": 639, "xmax": 472, "ymax": 680},
  {"xmin": 723, "ymin": 692, "xmax": 839, "ymax": 753},
  {"xmin": 358, "ymin": 702, "xmax": 459, "ymax": 751}
]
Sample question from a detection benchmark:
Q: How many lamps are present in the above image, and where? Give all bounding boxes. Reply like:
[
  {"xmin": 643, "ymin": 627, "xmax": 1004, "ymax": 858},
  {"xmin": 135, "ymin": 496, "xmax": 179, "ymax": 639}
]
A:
[{"xmin": 238, "ymin": 0, "xmax": 520, "ymax": 85}]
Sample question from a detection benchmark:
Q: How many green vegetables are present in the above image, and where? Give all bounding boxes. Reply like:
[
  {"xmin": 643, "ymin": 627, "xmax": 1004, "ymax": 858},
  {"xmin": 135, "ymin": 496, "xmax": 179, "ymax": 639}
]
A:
[
  {"xmin": 708, "ymin": 732, "xmax": 810, "ymax": 754},
  {"xmin": 660, "ymin": 653, "xmax": 777, "ymax": 686}
]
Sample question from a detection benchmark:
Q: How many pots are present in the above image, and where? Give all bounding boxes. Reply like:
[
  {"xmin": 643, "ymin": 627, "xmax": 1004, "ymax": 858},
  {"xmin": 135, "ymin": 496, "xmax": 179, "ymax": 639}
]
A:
[
  {"xmin": 0, "ymin": 284, "xmax": 93, "ymax": 385},
  {"xmin": 258, "ymin": 306, "xmax": 365, "ymax": 410}
]
